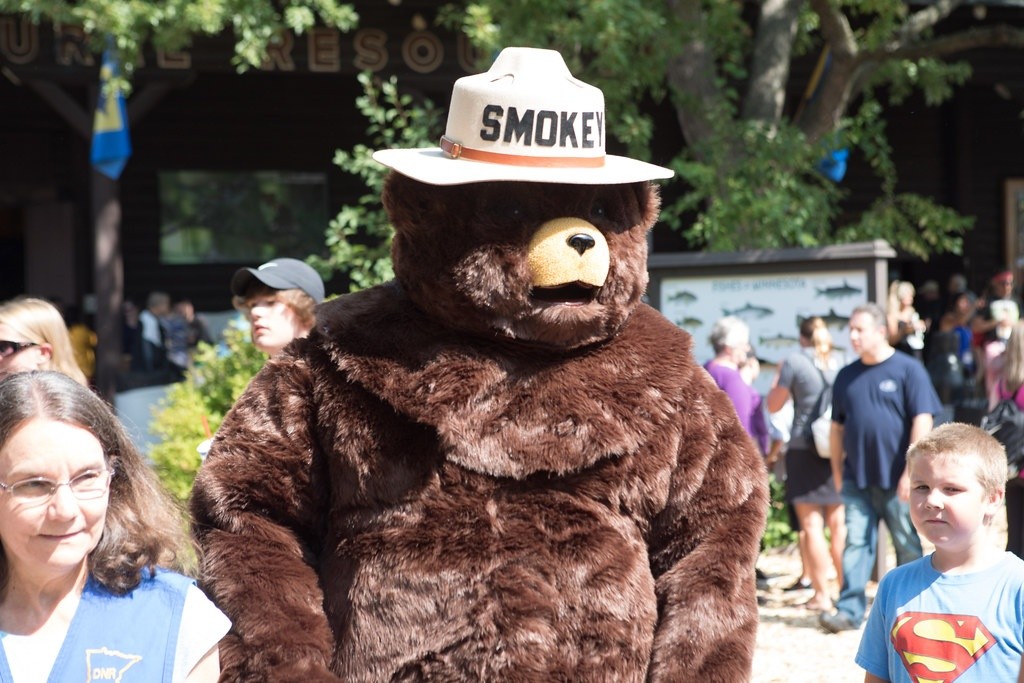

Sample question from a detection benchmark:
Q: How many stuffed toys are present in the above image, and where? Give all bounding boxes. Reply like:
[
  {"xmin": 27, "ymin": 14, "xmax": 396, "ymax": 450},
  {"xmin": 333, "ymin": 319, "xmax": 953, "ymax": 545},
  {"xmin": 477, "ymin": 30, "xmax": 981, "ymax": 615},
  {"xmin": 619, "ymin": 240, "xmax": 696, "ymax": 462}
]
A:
[{"xmin": 181, "ymin": 46, "xmax": 773, "ymax": 683}]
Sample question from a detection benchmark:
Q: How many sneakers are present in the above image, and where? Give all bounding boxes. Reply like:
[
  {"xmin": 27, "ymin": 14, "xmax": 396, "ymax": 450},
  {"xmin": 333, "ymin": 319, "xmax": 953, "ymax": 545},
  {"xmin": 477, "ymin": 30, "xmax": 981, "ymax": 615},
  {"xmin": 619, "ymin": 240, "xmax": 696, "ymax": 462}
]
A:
[{"xmin": 783, "ymin": 577, "xmax": 814, "ymax": 592}]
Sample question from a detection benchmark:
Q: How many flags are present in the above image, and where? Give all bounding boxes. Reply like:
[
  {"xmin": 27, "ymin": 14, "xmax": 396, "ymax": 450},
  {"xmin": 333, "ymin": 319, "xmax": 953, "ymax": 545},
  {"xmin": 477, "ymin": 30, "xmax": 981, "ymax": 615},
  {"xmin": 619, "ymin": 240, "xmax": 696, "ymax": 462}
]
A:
[{"xmin": 92, "ymin": 35, "xmax": 133, "ymax": 179}]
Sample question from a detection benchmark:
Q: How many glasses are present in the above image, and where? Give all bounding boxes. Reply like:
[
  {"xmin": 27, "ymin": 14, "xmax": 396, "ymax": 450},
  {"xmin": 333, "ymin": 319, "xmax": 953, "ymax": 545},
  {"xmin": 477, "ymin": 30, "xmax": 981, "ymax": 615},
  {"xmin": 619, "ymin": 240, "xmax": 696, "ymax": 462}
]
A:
[
  {"xmin": 1, "ymin": 469, "xmax": 115, "ymax": 504},
  {"xmin": 0, "ymin": 340, "xmax": 37, "ymax": 356}
]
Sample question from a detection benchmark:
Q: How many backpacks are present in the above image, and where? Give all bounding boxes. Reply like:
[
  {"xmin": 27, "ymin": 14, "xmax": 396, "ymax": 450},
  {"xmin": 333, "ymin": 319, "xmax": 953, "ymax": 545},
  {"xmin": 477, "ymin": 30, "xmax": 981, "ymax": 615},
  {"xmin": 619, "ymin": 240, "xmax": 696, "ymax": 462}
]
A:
[{"xmin": 801, "ymin": 348, "xmax": 847, "ymax": 460}]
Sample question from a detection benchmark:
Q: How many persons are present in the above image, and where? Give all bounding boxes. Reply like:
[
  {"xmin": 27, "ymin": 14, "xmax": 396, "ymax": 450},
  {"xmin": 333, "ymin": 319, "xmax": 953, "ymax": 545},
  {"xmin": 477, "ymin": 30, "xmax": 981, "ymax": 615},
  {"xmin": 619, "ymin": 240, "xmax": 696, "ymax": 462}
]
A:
[
  {"xmin": 0, "ymin": 372, "xmax": 235, "ymax": 683},
  {"xmin": 700, "ymin": 315, "xmax": 768, "ymax": 606},
  {"xmin": 731, "ymin": 353, "xmax": 789, "ymax": 471},
  {"xmin": 63, "ymin": 288, "xmax": 217, "ymax": 386},
  {"xmin": 884, "ymin": 269, "xmax": 1024, "ymax": 481},
  {"xmin": 818, "ymin": 302, "xmax": 945, "ymax": 632},
  {"xmin": 854, "ymin": 420, "xmax": 1024, "ymax": 683},
  {"xmin": 232, "ymin": 256, "xmax": 328, "ymax": 361},
  {"xmin": 769, "ymin": 313, "xmax": 853, "ymax": 611},
  {"xmin": 0, "ymin": 294, "xmax": 88, "ymax": 387}
]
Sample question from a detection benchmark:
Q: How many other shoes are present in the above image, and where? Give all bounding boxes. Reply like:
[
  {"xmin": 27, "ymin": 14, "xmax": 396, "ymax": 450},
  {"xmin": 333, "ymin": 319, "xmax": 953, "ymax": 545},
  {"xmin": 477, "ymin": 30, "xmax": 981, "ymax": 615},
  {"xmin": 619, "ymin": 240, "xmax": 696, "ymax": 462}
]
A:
[{"xmin": 819, "ymin": 610, "xmax": 857, "ymax": 634}]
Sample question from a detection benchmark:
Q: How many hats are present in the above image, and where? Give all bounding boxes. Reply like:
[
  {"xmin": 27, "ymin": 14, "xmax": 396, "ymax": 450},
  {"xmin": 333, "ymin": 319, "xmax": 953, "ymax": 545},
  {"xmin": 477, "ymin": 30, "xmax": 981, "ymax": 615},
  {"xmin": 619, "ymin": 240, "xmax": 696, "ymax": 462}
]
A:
[
  {"xmin": 230, "ymin": 257, "xmax": 325, "ymax": 304},
  {"xmin": 374, "ymin": 45, "xmax": 675, "ymax": 186}
]
